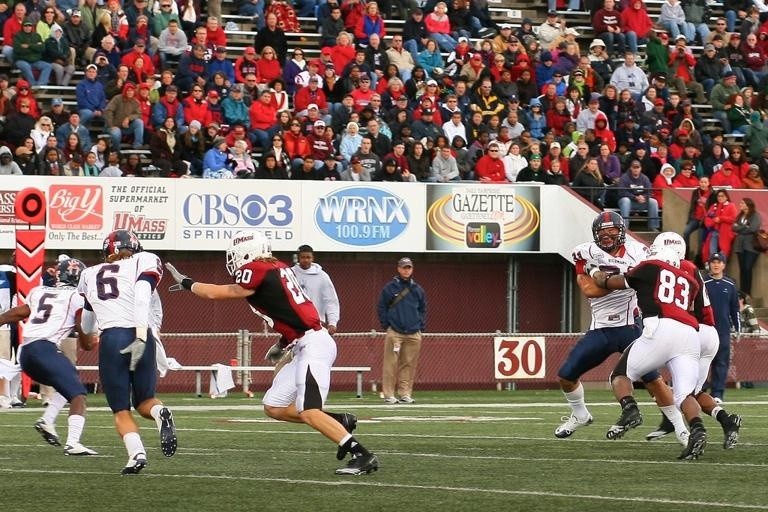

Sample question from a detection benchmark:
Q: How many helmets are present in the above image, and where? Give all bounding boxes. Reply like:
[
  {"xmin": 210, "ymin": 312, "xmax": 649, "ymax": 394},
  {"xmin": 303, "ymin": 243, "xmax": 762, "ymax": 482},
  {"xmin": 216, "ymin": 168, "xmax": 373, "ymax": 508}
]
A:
[
  {"xmin": 102, "ymin": 229, "xmax": 143, "ymax": 261},
  {"xmin": 226, "ymin": 230, "xmax": 272, "ymax": 276},
  {"xmin": 591, "ymin": 210, "xmax": 627, "ymax": 253},
  {"xmin": 644, "ymin": 231, "xmax": 687, "ymax": 269},
  {"xmin": 54, "ymin": 258, "xmax": 87, "ymax": 287}
]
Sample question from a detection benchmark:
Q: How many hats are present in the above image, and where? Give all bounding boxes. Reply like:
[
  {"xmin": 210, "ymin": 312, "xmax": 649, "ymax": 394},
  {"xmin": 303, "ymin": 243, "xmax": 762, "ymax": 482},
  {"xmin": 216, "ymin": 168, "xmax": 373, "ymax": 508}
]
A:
[
  {"xmin": 708, "ymin": 253, "xmax": 727, "ymax": 265},
  {"xmin": 657, "ymin": 33, "xmax": 668, "ymax": 39},
  {"xmin": 307, "ymin": 103, "xmax": 319, "ymax": 110},
  {"xmin": 722, "ymin": 71, "xmax": 737, "ymax": 80},
  {"xmin": 72, "ymin": 9, "xmax": 81, "ymax": 17},
  {"xmin": 500, "ymin": 8, "xmax": 585, "ymax": 76},
  {"xmin": 16, "ymin": 146, "xmax": 34, "ymax": 156},
  {"xmin": 703, "ymin": 34, "xmax": 741, "ymax": 55},
  {"xmin": 420, "ymin": 80, "xmax": 438, "ymax": 116},
  {"xmin": 313, "ymin": 119, "xmax": 325, "ymax": 127},
  {"xmin": 245, "ymin": 47, "xmax": 256, "ymax": 55},
  {"xmin": 122, "ymin": 82, "xmax": 178, "ymax": 97},
  {"xmin": 307, "ymin": 47, "xmax": 336, "ymax": 83},
  {"xmin": 189, "ymin": 120, "xmax": 248, "ymax": 153},
  {"xmin": 458, "ymin": 36, "xmax": 468, "ymax": 43},
  {"xmin": 398, "ymin": 257, "xmax": 413, "ymax": 268},
  {"xmin": 674, "ymin": 34, "xmax": 687, "ymax": 44},
  {"xmin": 624, "ymin": 98, "xmax": 760, "ymax": 171},
  {"xmin": 508, "ymin": 93, "xmax": 519, "ymax": 101},
  {"xmin": 320, "ymin": 152, "xmax": 340, "ymax": 162},
  {"xmin": 51, "ymin": 98, "xmax": 63, "ymax": 107},
  {"xmin": 207, "ymin": 90, "xmax": 219, "ymax": 97},
  {"xmin": 529, "ymin": 141, "xmax": 560, "ymax": 160}
]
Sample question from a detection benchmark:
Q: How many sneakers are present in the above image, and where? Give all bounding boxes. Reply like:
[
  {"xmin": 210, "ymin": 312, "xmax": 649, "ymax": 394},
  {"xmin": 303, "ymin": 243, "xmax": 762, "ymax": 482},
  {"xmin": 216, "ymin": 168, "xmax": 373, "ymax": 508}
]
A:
[
  {"xmin": 335, "ymin": 453, "xmax": 378, "ymax": 476},
  {"xmin": 33, "ymin": 417, "xmax": 62, "ymax": 446},
  {"xmin": 645, "ymin": 410, "xmax": 743, "ymax": 461},
  {"xmin": 63, "ymin": 442, "xmax": 99, "ymax": 456},
  {"xmin": 336, "ymin": 413, "xmax": 357, "ymax": 460},
  {"xmin": 0, "ymin": 395, "xmax": 49, "ymax": 409},
  {"xmin": 120, "ymin": 451, "xmax": 148, "ymax": 474},
  {"xmin": 384, "ymin": 395, "xmax": 416, "ymax": 404},
  {"xmin": 606, "ymin": 407, "xmax": 643, "ymax": 440},
  {"xmin": 155, "ymin": 407, "xmax": 177, "ymax": 457},
  {"xmin": 554, "ymin": 411, "xmax": 594, "ymax": 438}
]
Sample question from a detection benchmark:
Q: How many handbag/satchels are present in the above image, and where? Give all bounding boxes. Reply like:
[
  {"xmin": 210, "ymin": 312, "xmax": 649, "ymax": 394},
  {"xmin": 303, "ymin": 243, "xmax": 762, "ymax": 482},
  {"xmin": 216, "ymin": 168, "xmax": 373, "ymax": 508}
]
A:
[{"xmin": 752, "ymin": 230, "xmax": 768, "ymax": 253}]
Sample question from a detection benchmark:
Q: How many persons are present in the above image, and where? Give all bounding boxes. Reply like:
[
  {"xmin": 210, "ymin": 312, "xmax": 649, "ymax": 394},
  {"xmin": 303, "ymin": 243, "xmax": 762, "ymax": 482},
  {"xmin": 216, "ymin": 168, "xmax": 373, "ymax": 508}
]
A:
[
  {"xmin": 0, "ymin": 257, "xmax": 99, "ymax": 455},
  {"xmin": 645, "ymin": 231, "xmax": 742, "ymax": 449},
  {"xmin": 738, "ymin": 291, "xmax": 761, "ymax": 388},
  {"xmin": 555, "ymin": 211, "xmax": 691, "ymax": 448},
  {"xmin": 0, "ymin": 0, "xmax": 768, "ymax": 296},
  {"xmin": 378, "ymin": 258, "xmax": 428, "ymax": 403},
  {"xmin": 583, "ymin": 247, "xmax": 706, "ymax": 461},
  {"xmin": 290, "ymin": 245, "xmax": 340, "ymax": 335},
  {"xmin": 700, "ymin": 252, "xmax": 741, "ymax": 403},
  {"xmin": 165, "ymin": 230, "xmax": 378, "ymax": 476},
  {"xmin": 77, "ymin": 230, "xmax": 177, "ymax": 473}
]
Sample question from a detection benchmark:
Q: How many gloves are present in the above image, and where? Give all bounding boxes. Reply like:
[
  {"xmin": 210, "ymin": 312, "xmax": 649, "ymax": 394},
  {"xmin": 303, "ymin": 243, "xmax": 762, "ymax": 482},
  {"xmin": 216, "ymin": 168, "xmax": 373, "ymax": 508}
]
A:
[
  {"xmin": 120, "ymin": 337, "xmax": 147, "ymax": 371},
  {"xmin": 165, "ymin": 261, "xmax": 192, "ymax": 292},
  {"xmin": 582, "ymin": 258, "xmax": 600, "ymax": 280},
  {"xmin": 264, "ymin": 343, "xmax": 286, "ymax": 365}
]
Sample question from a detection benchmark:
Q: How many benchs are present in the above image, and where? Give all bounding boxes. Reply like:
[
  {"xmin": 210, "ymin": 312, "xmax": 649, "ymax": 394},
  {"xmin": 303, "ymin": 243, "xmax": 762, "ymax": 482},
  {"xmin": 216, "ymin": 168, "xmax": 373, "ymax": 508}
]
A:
[
  {"xmin": 0, "ymin": 1, "xmax": 768, "ymax": 231},
  {"xmin": 73, "ymin": 364, "xmax": 373, "ymax": 399}
]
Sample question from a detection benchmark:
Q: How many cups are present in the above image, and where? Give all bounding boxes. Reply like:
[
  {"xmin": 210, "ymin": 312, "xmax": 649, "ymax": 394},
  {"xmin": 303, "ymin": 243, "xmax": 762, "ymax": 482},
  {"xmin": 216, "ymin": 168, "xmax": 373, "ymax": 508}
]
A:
[{"xmin": 229, "ymin": 359, "xmax": 238, "ymax": 366}]
